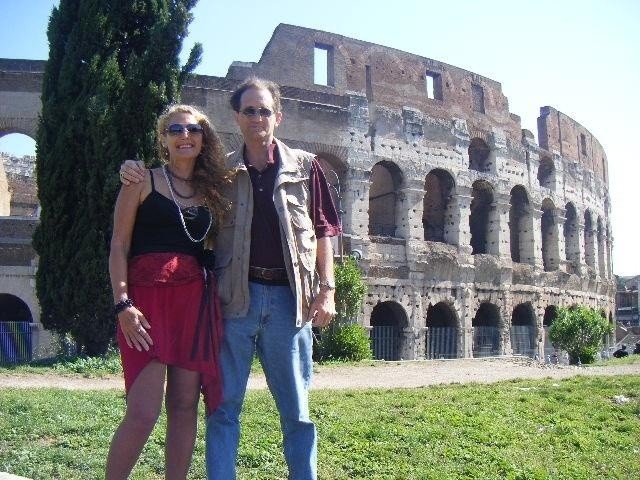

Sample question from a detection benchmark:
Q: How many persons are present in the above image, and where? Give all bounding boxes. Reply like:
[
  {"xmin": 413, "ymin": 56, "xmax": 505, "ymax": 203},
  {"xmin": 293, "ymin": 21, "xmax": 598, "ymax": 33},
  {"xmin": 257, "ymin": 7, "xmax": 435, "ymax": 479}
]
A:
[
  {"xmin": 633, "ymin": 342, "xmax": 640, "ymax": 354},
  {"xmin": 613, "ymin": 344, "xmax": 629, "ymax": 358}
]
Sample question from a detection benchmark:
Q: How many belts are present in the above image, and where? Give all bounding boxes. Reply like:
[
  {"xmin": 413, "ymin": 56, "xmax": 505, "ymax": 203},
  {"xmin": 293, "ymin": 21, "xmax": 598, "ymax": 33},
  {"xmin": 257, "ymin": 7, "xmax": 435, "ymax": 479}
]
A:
[{"xmin": 248, "ymin": 266, "xmax": 289, "ymax": 284}]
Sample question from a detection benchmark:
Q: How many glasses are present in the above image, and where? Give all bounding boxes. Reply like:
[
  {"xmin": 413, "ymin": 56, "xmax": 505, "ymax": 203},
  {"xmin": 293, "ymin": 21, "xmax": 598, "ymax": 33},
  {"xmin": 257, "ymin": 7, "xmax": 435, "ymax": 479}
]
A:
[
  {"xmin": 162, "ymin": 124, "xmax": 204, "ymax": 137},
  {"xmin": 238, "ymin": 105, "xmax": 275, "ymax": 117}
]
